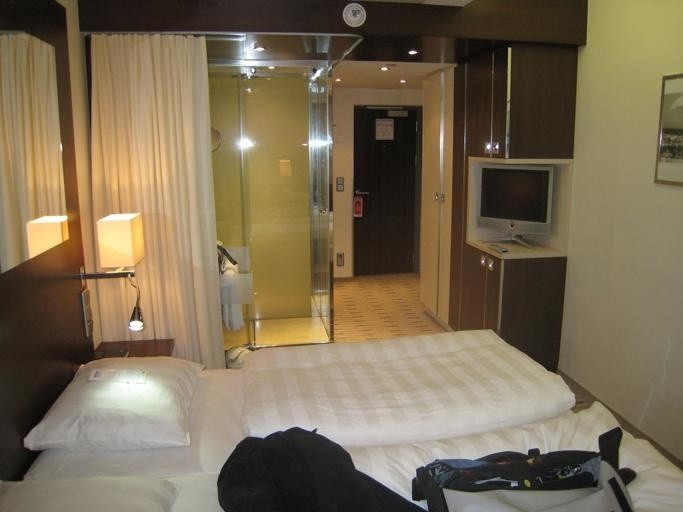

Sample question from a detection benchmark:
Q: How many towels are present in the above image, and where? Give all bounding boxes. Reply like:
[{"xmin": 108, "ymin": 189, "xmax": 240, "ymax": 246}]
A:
[{"xmin": 220, "ymin": 259, "xmax": 245, "ymax": 331}]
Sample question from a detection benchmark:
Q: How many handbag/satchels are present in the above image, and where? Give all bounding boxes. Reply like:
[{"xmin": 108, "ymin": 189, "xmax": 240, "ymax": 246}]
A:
[{"xmin": 427, "ymin": 447, "xmax": 634, "ymax": 512}]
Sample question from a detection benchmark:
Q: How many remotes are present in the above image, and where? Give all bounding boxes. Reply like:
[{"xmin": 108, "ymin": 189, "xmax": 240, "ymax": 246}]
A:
[{"xmin": 488, "ymin": 245, "xmax": 509, "ymax": 254}]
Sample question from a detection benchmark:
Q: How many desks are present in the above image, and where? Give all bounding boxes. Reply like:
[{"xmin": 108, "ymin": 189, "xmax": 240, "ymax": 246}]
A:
[{"xmin": 94, "ymin": 339, "xmax": 175, "ymax": 359}]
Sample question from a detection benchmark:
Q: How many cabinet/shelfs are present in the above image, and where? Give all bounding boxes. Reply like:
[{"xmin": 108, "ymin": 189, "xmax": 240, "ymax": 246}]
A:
[
  {"xmin": 449, "ymin": 65, "xmax": 469, "ymax": 332},
  {"xmin": 467, "ymin": 38, "xmax": 576, "ymax": 164},
  {"xmin": 460, "ymin": 243, "xmax": 568, "ymax": 372},
  {"xmin": 420, "ymin": 67, "xmax": 453, "ymax": 325}
]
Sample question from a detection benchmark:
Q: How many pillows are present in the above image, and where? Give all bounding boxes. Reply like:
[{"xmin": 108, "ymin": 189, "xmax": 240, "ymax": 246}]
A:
[{"xmin": 2, "ymin": 353, "xmax": 205, "ymax": 512}]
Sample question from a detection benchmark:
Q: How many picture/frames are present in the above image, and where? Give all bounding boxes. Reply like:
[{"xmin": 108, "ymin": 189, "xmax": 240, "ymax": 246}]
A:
[{"xmin": 653, "ymin": 72, "xmax": 682, "ymax": 186}]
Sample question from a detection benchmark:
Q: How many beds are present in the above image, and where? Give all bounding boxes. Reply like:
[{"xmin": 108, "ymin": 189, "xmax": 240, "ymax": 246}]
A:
[{"xmin": 2, "ymin": 330, "xmax": 681, "ymax": 511}]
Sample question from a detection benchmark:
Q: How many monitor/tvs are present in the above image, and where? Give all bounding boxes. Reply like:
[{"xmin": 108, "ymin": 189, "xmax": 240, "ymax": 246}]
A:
[{"xmin": 468, "ymin": 162, "xmax": 554, "ymax": 250}]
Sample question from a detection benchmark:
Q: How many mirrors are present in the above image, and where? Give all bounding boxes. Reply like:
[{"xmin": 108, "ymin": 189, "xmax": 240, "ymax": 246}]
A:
[{"xmin": 0, "ymin": 24, "xmax": 71, "ymax": 276}]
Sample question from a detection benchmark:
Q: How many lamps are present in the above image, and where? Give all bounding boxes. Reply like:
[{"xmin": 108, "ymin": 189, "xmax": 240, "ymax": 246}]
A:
[
  {"xmin": 26, "ymin": 214, "xmax": 67, "ymax": 260},
  {"xmin": 78, "ymin": 208, "xmax": 146, "ymax": 329}
]
